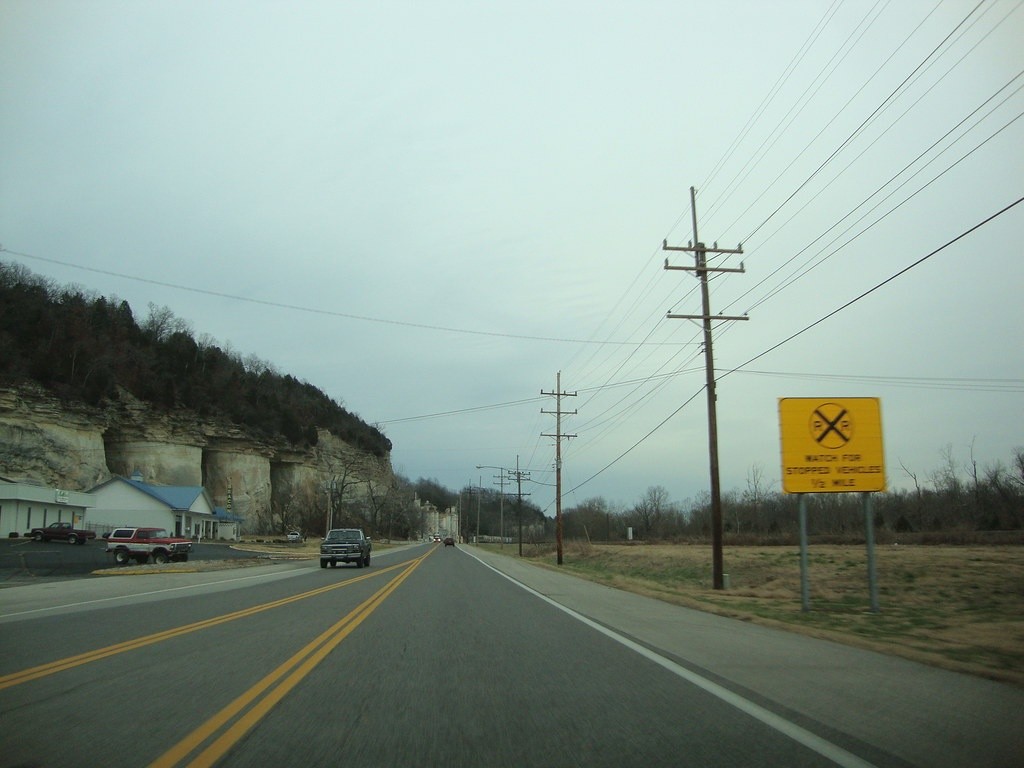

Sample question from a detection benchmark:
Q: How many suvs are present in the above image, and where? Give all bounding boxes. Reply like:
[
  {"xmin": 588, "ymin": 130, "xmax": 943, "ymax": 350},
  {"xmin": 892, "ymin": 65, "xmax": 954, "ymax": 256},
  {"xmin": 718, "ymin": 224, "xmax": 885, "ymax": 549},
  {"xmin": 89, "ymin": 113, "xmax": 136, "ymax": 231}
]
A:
[{"xmin": 103, "ymin": 527, "xmax": 192, "ymax": 565}]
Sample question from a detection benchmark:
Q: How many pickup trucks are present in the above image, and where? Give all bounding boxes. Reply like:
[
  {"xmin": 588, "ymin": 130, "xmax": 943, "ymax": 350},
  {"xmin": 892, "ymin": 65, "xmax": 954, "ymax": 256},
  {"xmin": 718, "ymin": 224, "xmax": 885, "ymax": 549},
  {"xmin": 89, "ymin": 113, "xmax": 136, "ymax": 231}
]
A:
[
  {"xmin": 287, "ymin": 531, "xmax": 300, "ymax": 541},
  {"xmin": 31, "ymin": 522, "xmax": 96, "ymax": 545},
  {"xmin": 319, "ymin": 528, "xmax": 372, "ymax": 568}
]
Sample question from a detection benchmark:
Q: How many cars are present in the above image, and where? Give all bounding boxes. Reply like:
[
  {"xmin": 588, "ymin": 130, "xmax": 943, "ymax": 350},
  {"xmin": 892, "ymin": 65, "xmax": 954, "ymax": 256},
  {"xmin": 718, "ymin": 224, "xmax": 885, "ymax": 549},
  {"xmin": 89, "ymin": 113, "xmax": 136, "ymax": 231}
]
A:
[
  {"xmin": 444, "ymin": 538, "xmax": 455, "ymax": 547},
  {"xmin": 434, "ymin": 537, "xmax": 441, "ymax": 542}
]
